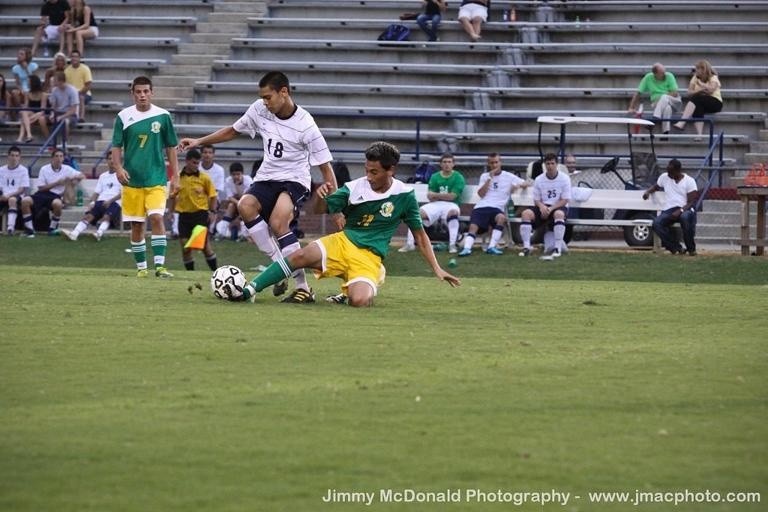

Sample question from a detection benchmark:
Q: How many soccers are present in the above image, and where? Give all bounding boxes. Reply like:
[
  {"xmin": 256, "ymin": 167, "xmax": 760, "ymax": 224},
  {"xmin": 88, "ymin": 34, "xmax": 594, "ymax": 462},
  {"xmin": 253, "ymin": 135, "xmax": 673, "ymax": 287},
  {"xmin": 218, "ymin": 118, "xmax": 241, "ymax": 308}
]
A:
[{"xmin": 211, "ymin": 265, "xmax": 247, "ymax": 298}]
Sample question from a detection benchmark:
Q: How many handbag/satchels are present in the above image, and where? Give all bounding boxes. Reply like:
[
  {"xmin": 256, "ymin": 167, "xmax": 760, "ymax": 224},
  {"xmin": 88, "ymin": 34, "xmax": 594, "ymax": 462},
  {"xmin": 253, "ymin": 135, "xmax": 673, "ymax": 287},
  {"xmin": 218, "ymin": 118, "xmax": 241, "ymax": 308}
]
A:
[
  {"xmin": 377, "ymin": 24, "xmax": 411, "ymax": 47},
  {"xmin": 744, "ymin": 175, "xmax": 768, "ymax": 188},
  {"xmin": 413, "ymin": 164, "xmax": 436, "ymax": 182}
]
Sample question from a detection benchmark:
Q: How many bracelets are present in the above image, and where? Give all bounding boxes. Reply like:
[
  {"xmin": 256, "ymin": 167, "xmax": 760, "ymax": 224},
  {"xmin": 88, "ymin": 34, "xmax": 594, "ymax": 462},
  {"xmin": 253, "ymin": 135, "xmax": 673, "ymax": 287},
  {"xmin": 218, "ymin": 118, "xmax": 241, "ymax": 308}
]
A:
[{"xmin": 680, "ymin": 208, "xmax": 685, "ymax": 213}]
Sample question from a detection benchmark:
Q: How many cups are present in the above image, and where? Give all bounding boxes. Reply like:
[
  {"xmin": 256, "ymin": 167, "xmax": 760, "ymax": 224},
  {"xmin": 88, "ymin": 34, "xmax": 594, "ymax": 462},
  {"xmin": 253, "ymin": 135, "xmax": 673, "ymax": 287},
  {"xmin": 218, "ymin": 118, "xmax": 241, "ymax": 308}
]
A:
[{"xmin": 231, "ymin": 226, "xmax": 238, "ymax": 240}]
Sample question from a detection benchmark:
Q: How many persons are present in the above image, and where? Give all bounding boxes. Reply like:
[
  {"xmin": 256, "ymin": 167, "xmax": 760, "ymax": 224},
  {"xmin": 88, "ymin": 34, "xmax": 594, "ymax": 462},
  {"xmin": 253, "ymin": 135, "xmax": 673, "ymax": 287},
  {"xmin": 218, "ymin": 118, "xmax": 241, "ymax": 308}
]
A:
[
  {"xmin": 174, "ymin": 70, "xmax": 347, "ymax": 304},
  {"xmin": 627, "ymin": 64, "xmax": 682, "ymax": 142},
  {"xmin": 643, "ymin": 159, "xmax": 699, "ymax": 256},
  {"xmin": 673, "ymin": 59, "xmax": 723, "ymax": 134},
  {"xmin": 170, "ymin": 150, "xmax": 219, "ymax": 270},
  {"xmin": 458, "ymin": 1, "xmax": 490, "ymax": 43},
  {"xmin": 226, "ymin": 141, "xmax": 461, "ymax": 308},
  {"xmin": 397, "ymin": 153, "xmax": 580, "ymax": 257},
  {"xmin": 111, "ymin": 78, "xmax": 180, "ymax": 277},
  {"xmin": 0, "ymin": 145, "xmax": 253, "ymax": 242},
  {"xmin": 0, "ymin": 1, "xmax": 99, "ymax": 145},
  {"xmin": 413, "ymin": 0, "xmax": 447, "ymax": 40}
]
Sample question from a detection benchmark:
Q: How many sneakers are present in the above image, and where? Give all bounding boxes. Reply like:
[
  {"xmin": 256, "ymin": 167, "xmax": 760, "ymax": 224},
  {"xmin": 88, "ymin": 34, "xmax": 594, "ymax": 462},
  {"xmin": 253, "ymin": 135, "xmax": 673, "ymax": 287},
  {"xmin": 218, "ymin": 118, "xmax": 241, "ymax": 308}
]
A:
[
  {"xmin": 48, "ymin": 227, "xmax": 101, "ymax": 242},
  {"xmin": 518, "ymin": 246, "xmax": 532, "ymax": 256},
  {"xmin": 459, "ymin": 247, "xmax": 472, "ymax": 256},
  {"xmin": 280, "ymin": 287, "xmax": 315, "ymax": 303},
  {"xmin": 155, "ymin": 267, "xmax": 174, "ymax": 279},
  {"xmin": 673, "ymin": 244, "xmax": 697, "ymax": 256},
  {"xmin": 6, "ymin": 225, "xmax": 35, "ymax": 238},
  {"xmin": 398, "ymin": 243, "xmax": 415, "ymax": 253},
  {"xmin": 552, "ymin": 246, "xmax": 561, "ymax": 257},
  {"xmin": 231, "ymin": 295, "xmax": 246, "ymax": 302},
  {"xmin": 273, "ymin": 277, "xmax": 288, "ymax": 296},
  {"xmin": 214, "ymin": 233, "xmax": 247, "ymax": 242},
  {"xmin": 448, "ymin": 243, "xmax": 457, "ymax": 253},
  {"xmin": 137, "ymin": 270, "xmax": 149, "ymax": 279},
  {"xmin": 487, "ymin": 246, "xmax": 504, "ymax": 255},
  {"xmin": 325, "ymin": 293, "xmax": 347, "ymax": 304}
]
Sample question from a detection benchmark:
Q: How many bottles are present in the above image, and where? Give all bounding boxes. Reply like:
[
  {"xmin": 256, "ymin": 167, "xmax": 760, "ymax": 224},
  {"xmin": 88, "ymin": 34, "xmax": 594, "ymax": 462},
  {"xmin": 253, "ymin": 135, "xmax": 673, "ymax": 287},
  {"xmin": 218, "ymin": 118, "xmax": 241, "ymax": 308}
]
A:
[
  {"xmin": 576, "ymin": 16, "xmax": 580, "ymax": 29},
  {"xmin": 448, "ymin": 256, "xmax": 457, "ymax": 268},
  {"xmin": 634, "ymin": 113, "xmax": 640, "ymax": 134},
  {"xmin": 586, "ymin": 17, "xmax": 590, "ymax": 28},
  {"xmin": 76, "ymin": 184, "xmax": 84, "ymax": 206},
  {"xmin": 510, "ymin": 7, "xmax": 516, "ymax": 21},
  {"xmin": 44, "ymin": 39, "xmax": 48, "ymax": 57},
  {"xmin": 557, "ymin": 150, "xmax": 562, "ymax": 163},
  {"xmin": 508, "ymin": 199, "xmax": 515, "ymax": 217},
  {"xmin": 503, "ymin": 9, "xmax": 509, "ymax": 21}
]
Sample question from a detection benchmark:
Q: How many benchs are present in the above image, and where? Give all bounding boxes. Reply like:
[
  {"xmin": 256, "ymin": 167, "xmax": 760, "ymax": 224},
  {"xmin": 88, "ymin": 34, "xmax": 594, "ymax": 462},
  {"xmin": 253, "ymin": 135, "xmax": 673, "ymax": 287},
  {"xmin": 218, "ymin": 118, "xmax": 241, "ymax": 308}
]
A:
[
  {"xmin": 27, "ymin": 179, "xmax": 124, "ymax": 230},
  {"xmin": 403, "ymin": 184, "xmax": 666, "ymax": 252},
  {"xmin": 0, "ymin": 1, "xmax": 216, "ymax": 148}
]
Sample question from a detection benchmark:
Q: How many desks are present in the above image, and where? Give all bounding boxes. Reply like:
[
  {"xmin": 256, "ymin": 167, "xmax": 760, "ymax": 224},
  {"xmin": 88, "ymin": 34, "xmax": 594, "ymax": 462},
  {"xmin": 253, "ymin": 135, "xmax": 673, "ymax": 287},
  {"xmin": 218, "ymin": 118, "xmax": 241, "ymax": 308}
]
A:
[{"xmin": 737, "ymin": 186, "xmax": 767, "ymax": 255}]
